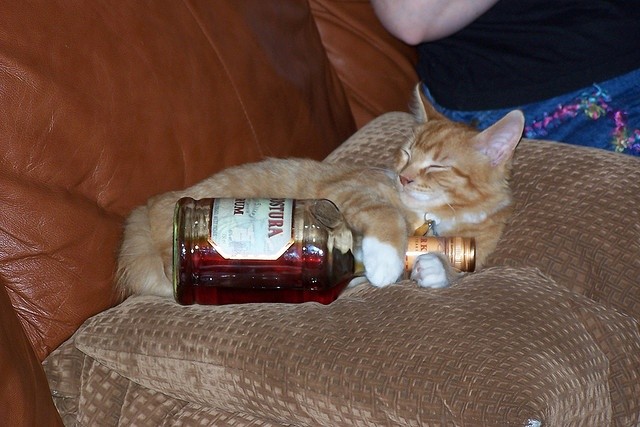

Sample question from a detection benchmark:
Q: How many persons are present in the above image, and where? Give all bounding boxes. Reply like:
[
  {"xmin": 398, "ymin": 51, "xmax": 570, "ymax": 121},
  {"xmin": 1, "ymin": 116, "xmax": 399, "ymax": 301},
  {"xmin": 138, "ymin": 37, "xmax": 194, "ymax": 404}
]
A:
[{"xmin": 369, "ymin": 0, "xmax": 640, "ymax": 157}]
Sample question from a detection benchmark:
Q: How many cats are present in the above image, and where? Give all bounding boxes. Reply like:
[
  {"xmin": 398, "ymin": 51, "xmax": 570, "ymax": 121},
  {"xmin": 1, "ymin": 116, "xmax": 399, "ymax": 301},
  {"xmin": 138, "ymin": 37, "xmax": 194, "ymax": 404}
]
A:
[{"xmin": 111, "ymin": 82, "xmax": 527, "ymax": 306}]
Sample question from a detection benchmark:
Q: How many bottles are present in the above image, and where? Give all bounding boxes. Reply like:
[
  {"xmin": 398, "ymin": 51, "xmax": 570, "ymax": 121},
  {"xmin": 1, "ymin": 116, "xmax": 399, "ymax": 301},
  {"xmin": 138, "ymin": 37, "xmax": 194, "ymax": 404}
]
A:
[{"xmin": 172, "ymin": 196, "xmax": 477, "ymax": 305}]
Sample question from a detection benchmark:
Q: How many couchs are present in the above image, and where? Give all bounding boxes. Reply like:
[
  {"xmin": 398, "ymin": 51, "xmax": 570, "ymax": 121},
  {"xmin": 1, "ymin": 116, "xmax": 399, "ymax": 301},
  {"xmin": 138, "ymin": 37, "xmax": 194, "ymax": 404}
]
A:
[{"xmin": 1, "ymin": 0, "xmax": 640, "ymax": 426}]
